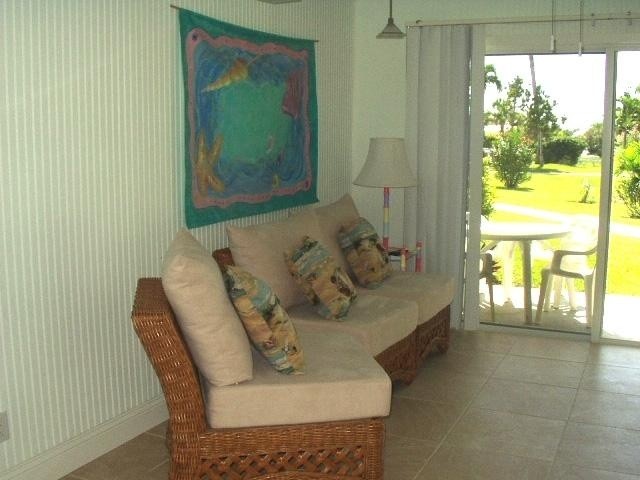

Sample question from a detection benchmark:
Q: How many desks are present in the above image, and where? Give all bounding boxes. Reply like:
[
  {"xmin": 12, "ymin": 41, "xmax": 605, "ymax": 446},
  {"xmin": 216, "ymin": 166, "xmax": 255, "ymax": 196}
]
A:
[{"xmin": 481, "ymin": 222, "xmax": 570, "ymax": 325}]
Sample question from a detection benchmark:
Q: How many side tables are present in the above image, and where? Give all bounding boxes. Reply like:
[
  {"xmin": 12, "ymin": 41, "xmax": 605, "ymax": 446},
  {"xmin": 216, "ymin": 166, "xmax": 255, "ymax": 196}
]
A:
[{"xmin": 389, "ymin": 240, "xmax": 423, "ymax": 272}]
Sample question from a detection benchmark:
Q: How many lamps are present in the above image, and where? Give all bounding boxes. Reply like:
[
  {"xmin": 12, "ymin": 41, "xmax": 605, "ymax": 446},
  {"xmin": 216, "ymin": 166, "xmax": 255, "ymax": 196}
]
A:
[
  {"xmin": 376, "ymin": 0, "xmax": 406, "ymax": 39},
  {"xmin": 352, "ymin": 138, "xmax": 419, "ymax": 254}
]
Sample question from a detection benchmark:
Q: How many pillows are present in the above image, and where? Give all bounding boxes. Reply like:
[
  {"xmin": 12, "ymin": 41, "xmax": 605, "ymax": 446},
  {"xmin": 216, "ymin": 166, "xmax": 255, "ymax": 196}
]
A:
[
  {"xmin": 162, "ymin": 229, "xmax": 254, "ymax": 387},
  {"xmin": 288, "ymin": 194, "xmax": 360, "ymax": 278},
  {"xmin": 336, "ymin": 217, "xmax": 393, "ymax": 289},
  {"xmin": 283, "ymin": 233, "xmax": 357, "ymax": 321},
  {"xmin": 226, "ymin": 264, "xmax": 306, "ymax": 376},
  {"xmin": 224, "ymin": 209, "xmax": 324, "ymax": 308}
]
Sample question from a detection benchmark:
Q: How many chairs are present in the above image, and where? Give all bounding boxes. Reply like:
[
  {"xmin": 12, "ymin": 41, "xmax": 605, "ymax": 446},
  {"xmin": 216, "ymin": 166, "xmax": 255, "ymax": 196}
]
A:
[
  {"xmin": 534, "ymin": 242, "xmax": 598, "ymax": 329},
  {"xmin": 464, "ymin": 212, "xmax": 495, "ymax": 321}
]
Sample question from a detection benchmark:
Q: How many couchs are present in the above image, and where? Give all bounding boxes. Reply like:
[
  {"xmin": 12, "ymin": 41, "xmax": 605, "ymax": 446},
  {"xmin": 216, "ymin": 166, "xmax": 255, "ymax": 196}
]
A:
[
  {"xmin": 358, "ymin": 270, "xmax": 456, "ymax": 368},
  {"xmin": 213, "ymin": 246, "xmax": 420, "ymax": 385},
  {"xmin": 130, "ymin": 276, "xmax": 393, "ymax": 480}
]
